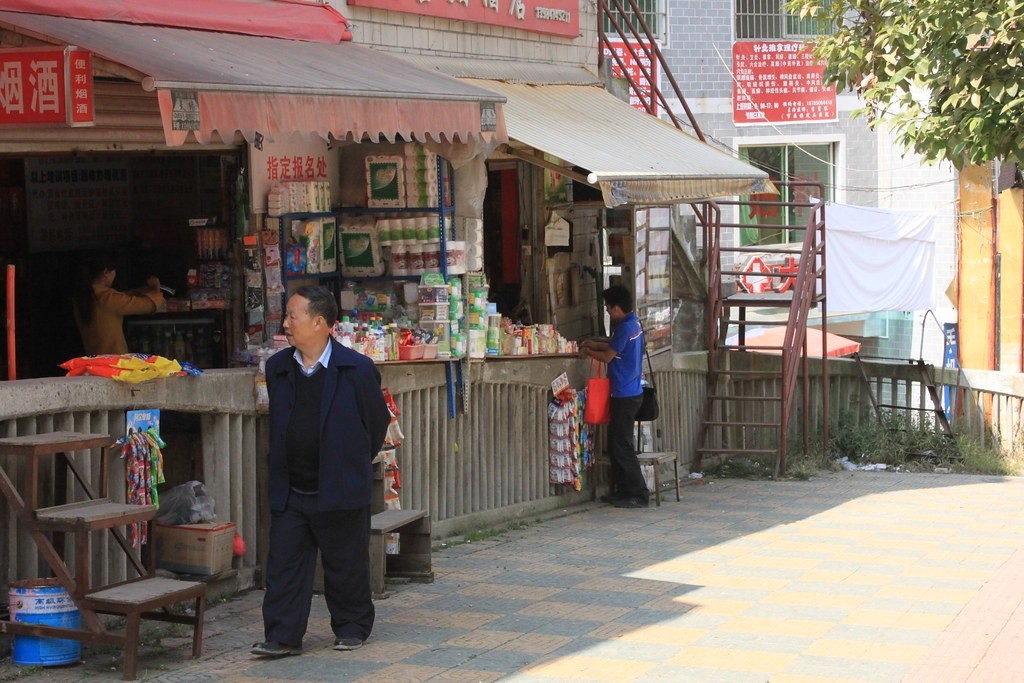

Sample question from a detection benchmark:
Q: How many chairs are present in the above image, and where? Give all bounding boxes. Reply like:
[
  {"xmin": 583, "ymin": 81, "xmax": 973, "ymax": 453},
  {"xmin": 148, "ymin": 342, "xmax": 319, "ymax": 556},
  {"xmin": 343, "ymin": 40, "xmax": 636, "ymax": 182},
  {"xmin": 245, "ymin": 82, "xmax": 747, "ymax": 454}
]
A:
[{"xmin": 615, "ymin": 418, "xmax": 680, "ymax": 507}]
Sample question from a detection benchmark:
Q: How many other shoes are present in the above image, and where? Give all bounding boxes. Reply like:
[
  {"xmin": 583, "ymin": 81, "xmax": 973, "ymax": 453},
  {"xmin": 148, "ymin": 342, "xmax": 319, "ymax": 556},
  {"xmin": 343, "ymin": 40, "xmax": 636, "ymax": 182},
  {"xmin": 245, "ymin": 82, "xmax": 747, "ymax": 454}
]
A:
[
  {"xmin": 614, "ymin": 489, "xmax": 649, "ymax": 508},
  {"xmin": 601, "ymin": 493, "xmax": 624, "ymax": 502},
  {"xmin": 250, "ymin": 639, "xmax": 302, "ymax": 655},
  {"xmin": 333, "ymin": 638, "xmax": 362, "ymax": 650}
]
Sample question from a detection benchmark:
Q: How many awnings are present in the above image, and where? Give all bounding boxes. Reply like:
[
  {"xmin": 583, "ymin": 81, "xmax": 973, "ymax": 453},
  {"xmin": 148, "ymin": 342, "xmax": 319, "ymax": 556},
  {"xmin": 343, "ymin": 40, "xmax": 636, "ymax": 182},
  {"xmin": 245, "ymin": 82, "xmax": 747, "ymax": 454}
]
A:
[
  {"xmin": 381, "ymin": 50, "xmax": 782, "ymax": 208},
  {"xmin": 0, "ymin": 11, "xmax": 507, "ymax": 148},
  {"xmin": 731, "ymin": 325, "xmax": 860, "ymax": 357}
]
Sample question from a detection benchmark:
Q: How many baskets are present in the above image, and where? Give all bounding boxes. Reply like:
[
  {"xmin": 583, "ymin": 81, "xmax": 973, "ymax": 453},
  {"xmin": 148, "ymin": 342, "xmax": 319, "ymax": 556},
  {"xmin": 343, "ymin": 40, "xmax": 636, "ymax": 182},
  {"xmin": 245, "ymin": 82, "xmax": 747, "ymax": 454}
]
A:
[
  {"xmin": 399, "ymin": 345, "xmax": 423, "ymax": 359},
  {"xmin": 423, "ymin": 345, "xmax": 437, "ymax": 359}
]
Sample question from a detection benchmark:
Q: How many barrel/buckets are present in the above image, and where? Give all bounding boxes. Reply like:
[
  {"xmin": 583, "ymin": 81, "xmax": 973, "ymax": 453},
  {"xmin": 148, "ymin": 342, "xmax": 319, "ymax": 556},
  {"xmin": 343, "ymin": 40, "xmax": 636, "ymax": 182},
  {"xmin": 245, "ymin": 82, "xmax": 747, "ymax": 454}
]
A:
[{"xmin": 5, "ymin": 577, "xmax": 83, "ymax": 666}]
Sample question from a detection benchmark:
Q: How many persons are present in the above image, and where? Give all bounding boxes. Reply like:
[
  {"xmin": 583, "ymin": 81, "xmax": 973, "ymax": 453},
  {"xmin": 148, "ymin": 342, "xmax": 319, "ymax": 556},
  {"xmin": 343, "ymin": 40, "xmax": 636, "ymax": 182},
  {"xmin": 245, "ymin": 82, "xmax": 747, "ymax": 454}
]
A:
[
  {"xmin": 578, "ymin": 285, "xmax": 649, "ymax": 508},
  {"xmin": 74, "ymin": 252, "xmax": 164, "ymax": 354},
  {"xmin": 250, "ymin": 283, "xmax": 391, "ymax": 655}
]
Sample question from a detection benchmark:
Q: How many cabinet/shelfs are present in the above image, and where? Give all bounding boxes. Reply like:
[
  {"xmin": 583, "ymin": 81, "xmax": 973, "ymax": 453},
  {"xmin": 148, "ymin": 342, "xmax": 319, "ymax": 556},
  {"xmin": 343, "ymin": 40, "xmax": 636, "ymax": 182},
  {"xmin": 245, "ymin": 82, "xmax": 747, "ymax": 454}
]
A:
[{"xmin": 124, "ymin": 317, "xmax": 216, "ymax": 371}]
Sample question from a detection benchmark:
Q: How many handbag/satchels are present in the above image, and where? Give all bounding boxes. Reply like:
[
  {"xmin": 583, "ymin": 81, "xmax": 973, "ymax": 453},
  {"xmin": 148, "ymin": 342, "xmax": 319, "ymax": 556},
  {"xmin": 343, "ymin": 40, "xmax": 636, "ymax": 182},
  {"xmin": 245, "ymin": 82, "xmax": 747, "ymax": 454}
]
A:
[
  {"xmin": 634, "ymin": 387, "xmax": 659, "ymax": 420},
  {"xmin": 584, "ymin": 352, "xmax": 609, "ymax": 424}
]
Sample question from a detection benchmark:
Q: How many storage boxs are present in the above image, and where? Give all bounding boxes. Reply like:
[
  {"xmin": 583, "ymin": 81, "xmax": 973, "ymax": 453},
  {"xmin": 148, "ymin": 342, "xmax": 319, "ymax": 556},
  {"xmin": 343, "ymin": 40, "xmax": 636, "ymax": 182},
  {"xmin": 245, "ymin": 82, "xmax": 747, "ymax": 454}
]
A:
[
  {"xmin": 608, "ymin": 232, "xmax": 634, "ymax": 266},
  {"xmin": 154, "ymin": 521, "xmax": 237, "ymax": 576}
]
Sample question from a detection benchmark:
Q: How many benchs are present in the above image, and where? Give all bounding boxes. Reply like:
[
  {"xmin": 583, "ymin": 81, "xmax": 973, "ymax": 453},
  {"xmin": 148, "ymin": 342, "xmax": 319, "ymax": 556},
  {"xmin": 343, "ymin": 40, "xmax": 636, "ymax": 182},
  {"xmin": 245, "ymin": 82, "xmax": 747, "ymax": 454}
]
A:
[{"xmin": 367, "ymin": 506, "xmax": 435, "ymax": 594}]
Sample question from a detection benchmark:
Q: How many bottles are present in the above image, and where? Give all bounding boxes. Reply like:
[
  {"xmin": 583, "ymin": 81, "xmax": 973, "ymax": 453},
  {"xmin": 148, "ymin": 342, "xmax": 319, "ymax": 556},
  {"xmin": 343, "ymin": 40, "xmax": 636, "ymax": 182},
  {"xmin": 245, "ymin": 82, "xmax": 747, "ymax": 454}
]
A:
[
  {"xmin": 141, "ymin": 326, "xmax": 209, "ymax": 364},
  {"xmin": 337, "ymin": 310, "xmax": 400, "ymax": 361}
]
